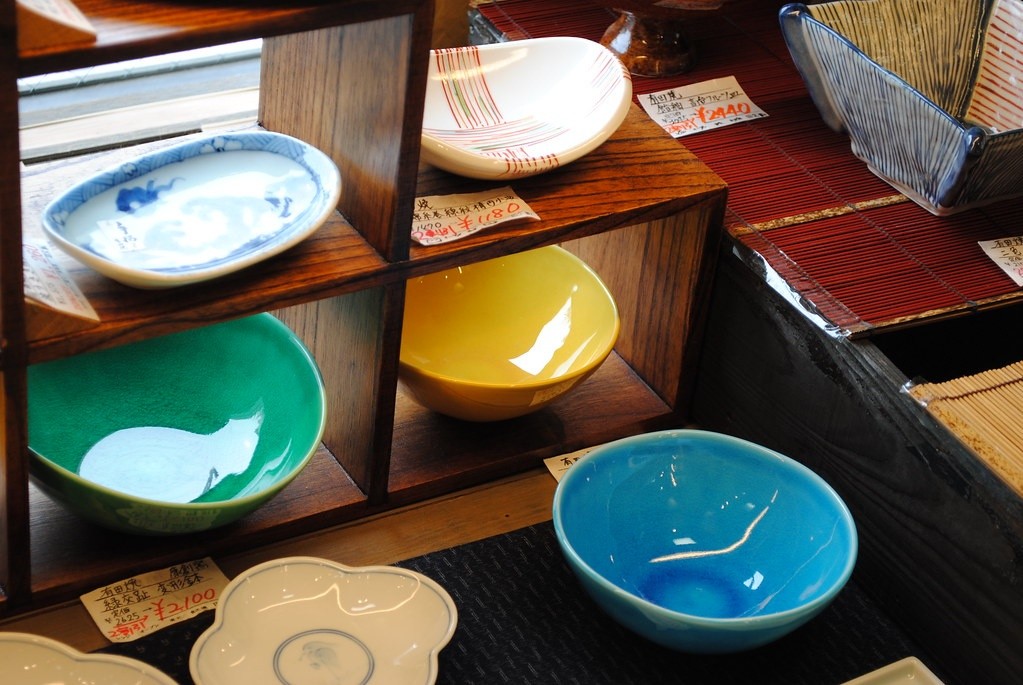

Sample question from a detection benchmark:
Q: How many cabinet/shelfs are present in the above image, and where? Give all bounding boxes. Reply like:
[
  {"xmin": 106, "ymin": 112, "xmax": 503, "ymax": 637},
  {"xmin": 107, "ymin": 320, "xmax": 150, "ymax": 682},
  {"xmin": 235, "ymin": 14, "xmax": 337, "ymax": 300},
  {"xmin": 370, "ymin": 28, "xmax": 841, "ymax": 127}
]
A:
[{"xmin": 0, "ymin": 0, "xmax": 731, "ymax": 567}]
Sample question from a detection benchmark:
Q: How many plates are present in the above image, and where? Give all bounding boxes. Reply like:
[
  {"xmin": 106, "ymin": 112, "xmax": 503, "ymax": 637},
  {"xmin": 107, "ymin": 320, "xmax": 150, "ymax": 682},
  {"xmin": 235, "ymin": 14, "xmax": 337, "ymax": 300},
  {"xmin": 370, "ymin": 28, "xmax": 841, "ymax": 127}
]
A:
[
  {"xmin": 836, "ymin": 656, "xmax": 947, "ymax": 685},
  {"xmin": 189, "ymin": 556, "xmax": 458, "ymax": 685},
  {"xmin": 421, "ymin": 37, "xmax": 632, "ymax": 180},
  {"xmin": 40, "ymin": 131, "xmax": 342, "ymax": 289}
]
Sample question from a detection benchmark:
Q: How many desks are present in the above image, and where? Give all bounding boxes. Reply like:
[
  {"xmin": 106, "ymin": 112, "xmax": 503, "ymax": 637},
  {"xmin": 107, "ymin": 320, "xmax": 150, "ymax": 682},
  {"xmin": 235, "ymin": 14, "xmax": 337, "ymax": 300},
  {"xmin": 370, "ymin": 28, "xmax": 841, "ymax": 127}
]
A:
[{"xmin": 0, "ymin": 412, "xmax": 957, "ymax": 685}]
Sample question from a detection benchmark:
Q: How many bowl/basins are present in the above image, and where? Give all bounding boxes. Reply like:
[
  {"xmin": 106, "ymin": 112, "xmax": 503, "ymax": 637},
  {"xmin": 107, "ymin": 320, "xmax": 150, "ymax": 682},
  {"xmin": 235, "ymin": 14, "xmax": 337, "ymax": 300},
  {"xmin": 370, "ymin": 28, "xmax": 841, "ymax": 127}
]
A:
[
  {"xmin": 0, "ymin": 632, "xmax": 177, "ymax": 685},
  {"xmin": 26, "ymin": 310, "xmax": 330, "ymax": 537},
  {"xmin": 397, "ymin": 245, "xmax": 619, "ymax": 422},
  {"xmin": 553, "ymin": 428, "xmax": 857, "ymax": 655},
  {"xmin": 779, "ymin": 0, "xmax": 1023, "ymax": 216}
]
